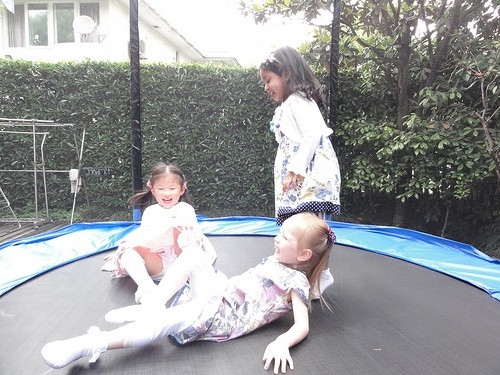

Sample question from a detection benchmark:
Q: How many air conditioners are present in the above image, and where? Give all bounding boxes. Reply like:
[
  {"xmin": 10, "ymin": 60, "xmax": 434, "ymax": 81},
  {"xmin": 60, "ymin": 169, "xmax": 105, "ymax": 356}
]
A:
[{"xmin": 127, "ymin": 37, "xmax": 148, "ymax": 60}]
